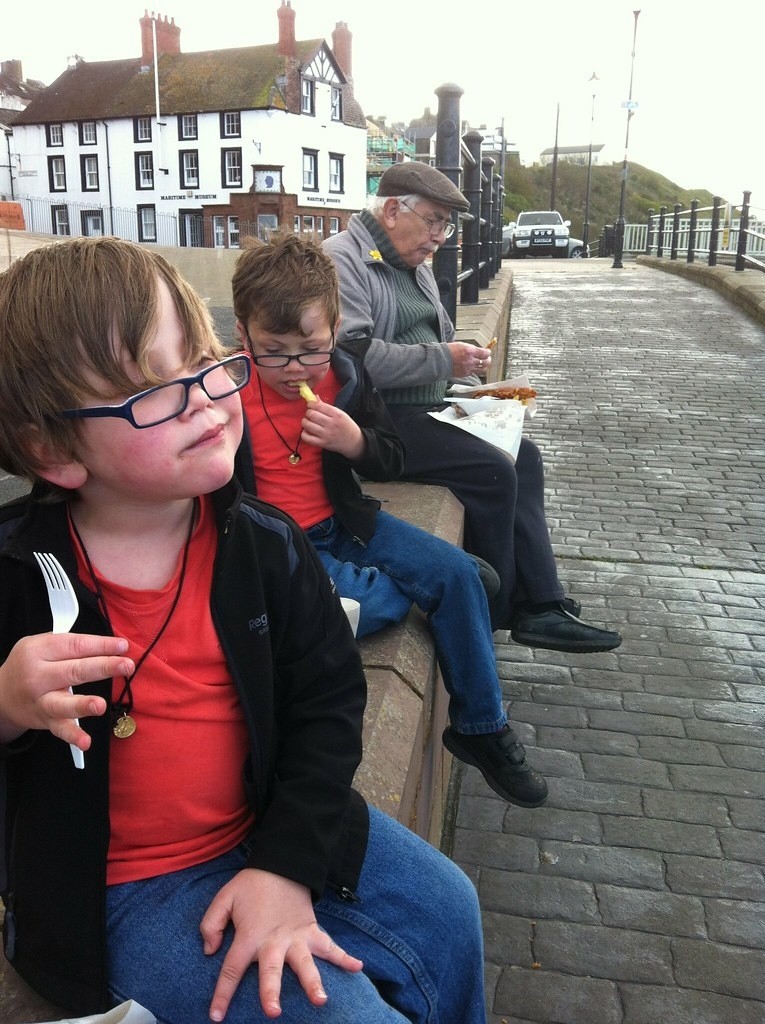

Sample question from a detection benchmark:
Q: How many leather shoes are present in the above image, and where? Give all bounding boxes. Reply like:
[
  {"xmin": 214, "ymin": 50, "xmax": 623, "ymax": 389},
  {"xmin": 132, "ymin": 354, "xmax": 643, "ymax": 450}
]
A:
[{"xmin": 499, "ymin": 596, "xmax": 622, "ymax": 653}]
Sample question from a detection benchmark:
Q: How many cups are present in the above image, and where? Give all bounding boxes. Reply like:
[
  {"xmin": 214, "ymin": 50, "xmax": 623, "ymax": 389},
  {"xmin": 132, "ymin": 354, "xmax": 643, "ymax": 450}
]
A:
[{"xmin": 339, "ymin": 597, "xmax": 361, "ymax": 639}]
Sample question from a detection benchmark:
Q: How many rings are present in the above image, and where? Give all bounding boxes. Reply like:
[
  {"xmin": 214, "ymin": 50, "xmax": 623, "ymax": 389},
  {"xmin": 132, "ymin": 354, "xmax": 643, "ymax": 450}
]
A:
[{"xmin": 479, "ymin": 359, "xmax": 483, "ymax": 367}]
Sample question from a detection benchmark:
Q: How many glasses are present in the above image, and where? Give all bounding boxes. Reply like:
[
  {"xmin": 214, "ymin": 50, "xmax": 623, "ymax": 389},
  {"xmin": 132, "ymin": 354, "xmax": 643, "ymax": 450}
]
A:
[
  {"xmin": 400, "ymin": 201, "xmax": 455, "ymax": 238},
  {"xmin": 50, "ymin": 353, "xmax": 250, "ymax": 429},
  {"xmin": 245, "ymin": 322, "xmax": 335, "ymax": 368}
]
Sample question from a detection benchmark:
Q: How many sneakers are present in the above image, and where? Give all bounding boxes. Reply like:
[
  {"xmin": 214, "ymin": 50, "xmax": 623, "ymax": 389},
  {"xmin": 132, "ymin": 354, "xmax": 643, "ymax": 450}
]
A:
[
  {"xmin": 442, "ymin": 722, "xmax": 549, "ymax": 808},
  {"xmin": 467, "ymin": 552, "xmax": 501, "ymax": 598}
]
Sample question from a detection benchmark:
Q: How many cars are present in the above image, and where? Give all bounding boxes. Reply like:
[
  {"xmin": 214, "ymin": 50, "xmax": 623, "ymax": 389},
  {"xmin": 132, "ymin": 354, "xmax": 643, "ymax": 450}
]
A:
[{"xmin": 502, "ymin": 224, "xmax": 590, "ymax": 258}]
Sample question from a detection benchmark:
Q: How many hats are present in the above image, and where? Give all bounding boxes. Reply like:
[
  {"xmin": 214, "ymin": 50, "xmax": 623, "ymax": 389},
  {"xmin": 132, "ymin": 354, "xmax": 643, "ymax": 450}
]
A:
[{"xmin": 377, "ymin": 162, "xmax": 471, "ymax": 213}]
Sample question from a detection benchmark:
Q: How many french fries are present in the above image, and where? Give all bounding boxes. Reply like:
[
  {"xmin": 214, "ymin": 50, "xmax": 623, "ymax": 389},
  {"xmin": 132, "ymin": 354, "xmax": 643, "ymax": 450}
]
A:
[{"xmin": 299, "ymin": 380, "xmax": 318, "ymax": 402}]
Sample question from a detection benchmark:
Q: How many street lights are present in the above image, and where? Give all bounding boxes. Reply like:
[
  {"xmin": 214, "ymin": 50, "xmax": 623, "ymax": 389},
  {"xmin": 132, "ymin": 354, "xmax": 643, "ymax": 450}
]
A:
[
  {"xmin": 611, "ymin": 0, "xmax": 642, "ymax": 268},
  {"xmin": 582, "ymin": 71, "xmax": 600, "ymax": 258},
  {"xmin": 550, "ymin": 89, "xmax": 563, "ymax": 211}
]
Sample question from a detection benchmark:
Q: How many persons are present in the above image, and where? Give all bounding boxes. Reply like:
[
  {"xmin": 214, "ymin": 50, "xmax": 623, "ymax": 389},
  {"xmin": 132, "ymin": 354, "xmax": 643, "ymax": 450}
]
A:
[
  {"xmin": 1, "ymin": 237, "xmax": 489, "ymax": 1024},
  {"xmin": 222, "ymin": 222, "xmax": 549, "ymax": 809},
  {"xmin": 318, "ymin": 161, "xmax": 623, "ymax": 654}
]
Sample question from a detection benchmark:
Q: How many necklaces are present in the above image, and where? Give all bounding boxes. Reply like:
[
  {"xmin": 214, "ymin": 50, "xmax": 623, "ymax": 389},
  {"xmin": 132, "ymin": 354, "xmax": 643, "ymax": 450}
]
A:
[
  {"xmin": 68, "ymin": 497, "xmax": 197, "ymax": 738},
  {"xmin": 257, "ymin": 372, "xmax": 313, "ymax": 465}
]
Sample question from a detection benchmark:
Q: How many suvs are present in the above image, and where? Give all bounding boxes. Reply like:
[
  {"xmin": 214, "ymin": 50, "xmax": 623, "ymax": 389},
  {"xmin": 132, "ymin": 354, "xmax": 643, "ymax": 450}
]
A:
[{"xmin": 509, "ymin": 210, "xmax": 571, "ymax": 258}]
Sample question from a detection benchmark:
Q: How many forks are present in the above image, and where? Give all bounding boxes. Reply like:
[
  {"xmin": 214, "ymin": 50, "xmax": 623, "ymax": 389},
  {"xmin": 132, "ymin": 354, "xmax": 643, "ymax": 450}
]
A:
[{"xmin": 33, "ymin": 551, "xmax": 85, "ymax": 771}]
